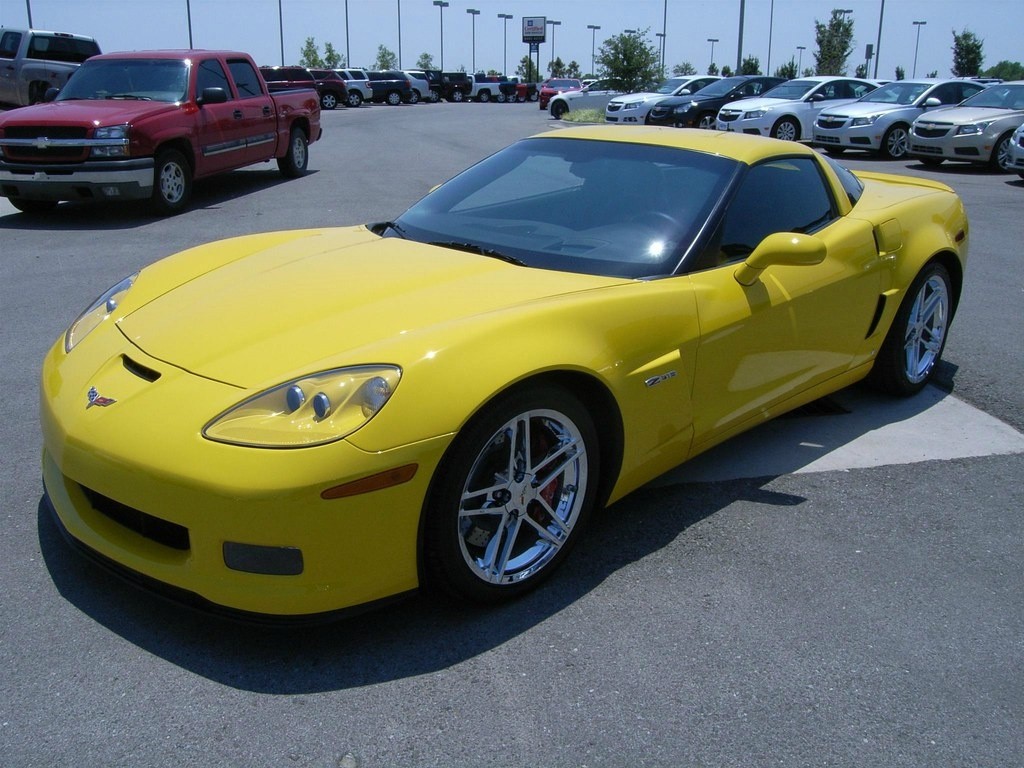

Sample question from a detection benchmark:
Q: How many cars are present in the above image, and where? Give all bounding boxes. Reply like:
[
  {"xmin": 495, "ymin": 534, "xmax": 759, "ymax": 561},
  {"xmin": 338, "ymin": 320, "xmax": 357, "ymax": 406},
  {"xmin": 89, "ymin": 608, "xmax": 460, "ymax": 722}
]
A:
[
  {"xmin": 905, "ymin": 80, "xmax": 1024, "ymax": 173},
  {"xmin": 547, "ymin": 77, "xmax": 658, "ymax": 119},
  {"xmin": 812, "ymin": 78, "xmax": 991, "ymax": 159},
  {"xmin": 540, "ymin": 78, "xmax": 587, "ymax": 109},
  {"xmin": 581, "ymin": 78, "xmax": 600, "ymax": 89},
  {"xmin": 1006, "ymin": 123, "xmax": 1024, "ymax": 180},
  {"xmin": 715, "ymin": 75, "xmax": 895, "ymax": 141},
  {"xmin": 649, "ymin": 74, "xmax": 789, "ymax": 129},
  {"xmin": 605, "ymin": 76, "xmax": 726, "ymax": 125}
]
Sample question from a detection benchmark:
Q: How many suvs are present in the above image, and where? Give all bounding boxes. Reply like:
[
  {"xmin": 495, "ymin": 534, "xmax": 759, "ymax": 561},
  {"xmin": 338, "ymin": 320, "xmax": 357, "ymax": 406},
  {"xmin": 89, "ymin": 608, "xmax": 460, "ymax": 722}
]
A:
[
  {"xmin": 258, "ymin": 65, "xmax": 317, "ymax": 93},
  {"xmin": 332, "ymin": 67, "xmax": 373, "ymax": 108},
  {"xmin": 420, "ymin": 70, "xmax": 443, "ymax": 102},
  {"xmin": 442, "ymin": 72, "xmax": 473, "ymax": 102},
  {"xmin": 364, "ymin": 70, "xmax": 413, "ymax": 105},
  {"xmin": 307, "ymin": 67, "xmax": 349, "ymax": 109},
  {"xmin": 399, "ymin": 70, "xmax": 430, "ymax": 104}
]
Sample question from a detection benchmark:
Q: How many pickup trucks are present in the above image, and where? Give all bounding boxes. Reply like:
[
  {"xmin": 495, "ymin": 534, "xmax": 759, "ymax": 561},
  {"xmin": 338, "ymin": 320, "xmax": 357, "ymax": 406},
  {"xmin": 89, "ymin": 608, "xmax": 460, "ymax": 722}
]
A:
[
  {"xmin": 476, "ymin": 74, "xmax": 540, "ymax": 102},
  {"xmin": 1, "ymin": 49, "xmax": 323, "ymax": 217},
  {"xmin": 1, "ymin": 27, "xmax": 103, "ymax": 107},
  {"xmin": 467, "ymin": 74, "xmax": 502, "ymax": 103}
]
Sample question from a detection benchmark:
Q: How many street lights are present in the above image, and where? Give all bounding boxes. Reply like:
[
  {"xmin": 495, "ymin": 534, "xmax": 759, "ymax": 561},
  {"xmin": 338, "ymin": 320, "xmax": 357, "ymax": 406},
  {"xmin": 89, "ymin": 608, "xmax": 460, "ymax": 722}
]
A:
[
  {"xmin": 625, "ymin": 30, "xmax": 636, "ymax": 36},
  {"xmin": 467, "ymin": 9, "xmax": 481, "ymax": 73},
  {"xmin": 913, "ymin": 21, "xmax": 927, "ymax": 78},
  {"xmin": 656, "ymin": 33, "xmax": 666, "ymax": 66},
  {"xmin": 587, "ymin": 25, "xmax": 602, "ymax": 77},
  {"xmin": 434, "ymin": 1, "xmax": 450, "ymax": 72},
  {"xmin": 708, "ymin": 39, "xmax": 719, "ymax": 64},
  {"xmin": 547, "ymin": 20, "xmax": 562, "ymax": 62},
  {"xmin": 797, "ymin": 47, "xmax": 806, "ymax": 77},
  {"xmin": 498, "ymin": 14, "xmax": 514, "ymax": 75}
]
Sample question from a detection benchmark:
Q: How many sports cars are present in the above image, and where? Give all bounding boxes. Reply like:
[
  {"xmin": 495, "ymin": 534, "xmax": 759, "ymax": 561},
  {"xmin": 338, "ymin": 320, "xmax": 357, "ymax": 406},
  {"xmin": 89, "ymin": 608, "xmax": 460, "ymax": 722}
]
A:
[{"xmin": 36, "ymin": 123, "xmax": 971, "ymax": 617}]
